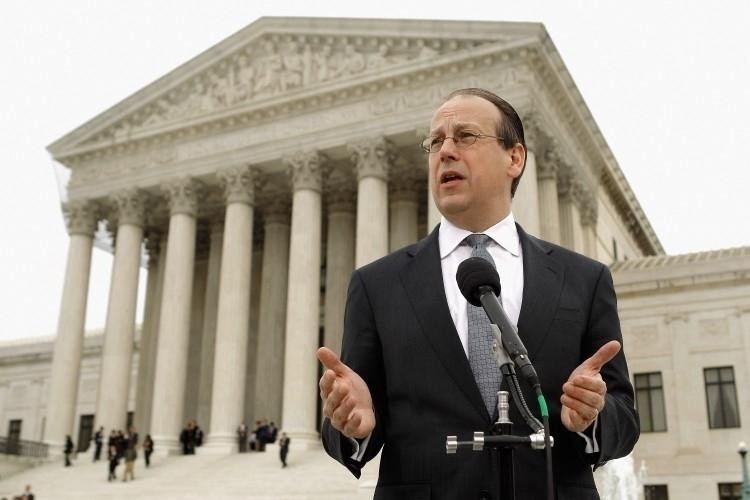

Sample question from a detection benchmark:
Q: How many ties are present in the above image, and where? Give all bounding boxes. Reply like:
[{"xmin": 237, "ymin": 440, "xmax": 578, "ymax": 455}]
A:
[{"xmin": 466, "ymin": 234, "xmax": 503, "ymax": 419}]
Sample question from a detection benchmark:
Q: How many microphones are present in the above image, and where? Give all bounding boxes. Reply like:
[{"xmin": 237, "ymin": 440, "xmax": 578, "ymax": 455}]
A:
[{"xmin": 455, "ymin": 256, "xmax": 542, "ymax": 386}]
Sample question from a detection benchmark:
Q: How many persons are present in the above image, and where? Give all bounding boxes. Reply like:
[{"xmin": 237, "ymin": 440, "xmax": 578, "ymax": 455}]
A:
[
  {"xmin": 0, "ymin": 416, "xmax": 293, "ymax": 500},
  {"xmin": 316, "ymin": 87, "xmax": 640, "ymax": 500}
]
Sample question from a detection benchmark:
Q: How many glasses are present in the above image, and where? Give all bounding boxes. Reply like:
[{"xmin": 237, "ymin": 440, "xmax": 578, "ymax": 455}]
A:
[{"xmin": 421, "ymin": 131, "xmax": 504, "ymax": 153}]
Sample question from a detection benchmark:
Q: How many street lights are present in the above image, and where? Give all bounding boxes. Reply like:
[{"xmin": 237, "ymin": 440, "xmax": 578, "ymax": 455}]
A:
[{"xmin": 736, "ymin": 441, "xmax": 750, "ymax": 500}]
